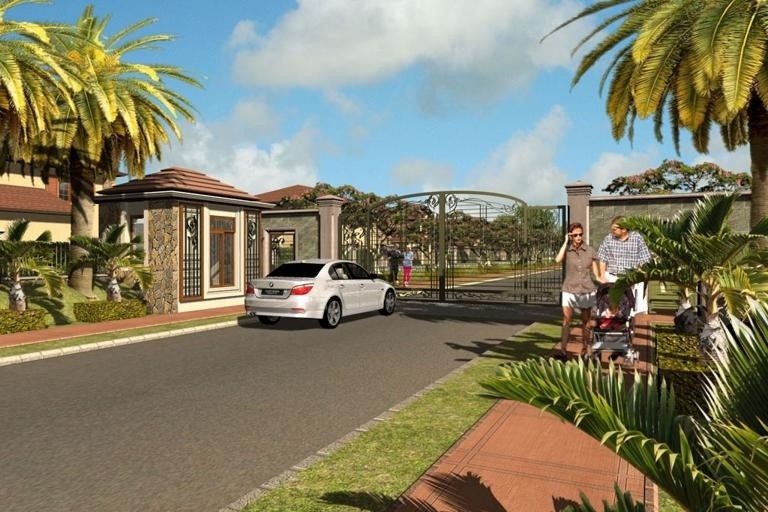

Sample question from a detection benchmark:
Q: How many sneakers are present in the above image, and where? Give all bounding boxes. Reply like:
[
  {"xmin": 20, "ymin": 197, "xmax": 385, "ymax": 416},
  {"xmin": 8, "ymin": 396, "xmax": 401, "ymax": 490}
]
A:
[{"xmin": 554, "ymin": 355, "xmax": 568, "ymax": 363}]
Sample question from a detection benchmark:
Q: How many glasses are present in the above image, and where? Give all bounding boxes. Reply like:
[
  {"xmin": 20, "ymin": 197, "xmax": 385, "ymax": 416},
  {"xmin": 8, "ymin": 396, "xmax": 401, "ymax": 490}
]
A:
[{"xmin": 573, "ymin": 233, "xmax": 584, "ymax": 236}]
